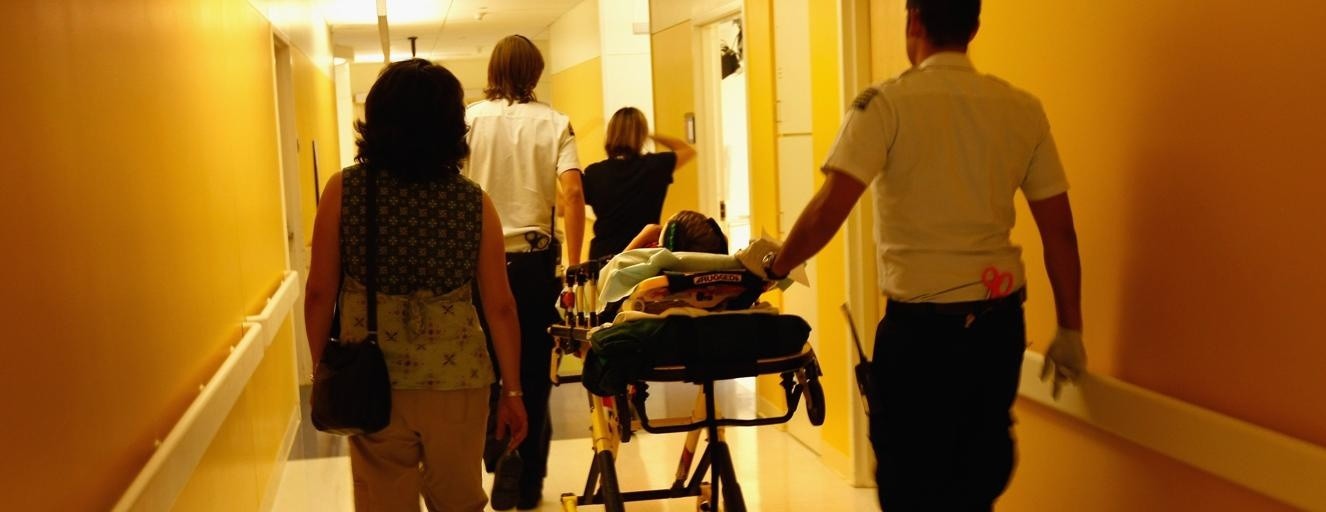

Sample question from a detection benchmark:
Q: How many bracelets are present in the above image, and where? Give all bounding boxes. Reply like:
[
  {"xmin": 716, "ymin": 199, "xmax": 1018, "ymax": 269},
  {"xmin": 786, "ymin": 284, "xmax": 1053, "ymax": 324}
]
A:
[{"xmin": 498, "ymin": 388, "xmax": 523, "ymax": 399}]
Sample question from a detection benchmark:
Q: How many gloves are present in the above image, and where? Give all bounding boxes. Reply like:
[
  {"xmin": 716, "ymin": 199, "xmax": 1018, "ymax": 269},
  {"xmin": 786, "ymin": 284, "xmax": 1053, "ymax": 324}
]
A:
[
  {"xmin": 736, "ymin": 236, "xmax": 782, "ymax": 286},
  {"xmin": 1032, "ymin": 329, "xmax": 1088, "ymax": 399}
]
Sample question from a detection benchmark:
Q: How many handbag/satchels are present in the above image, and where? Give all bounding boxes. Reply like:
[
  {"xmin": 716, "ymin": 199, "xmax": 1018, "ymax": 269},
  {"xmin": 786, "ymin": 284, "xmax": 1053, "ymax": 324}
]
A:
[{"xmin": 305, "ymin": 338, "xmax": 389, "ymax": 437}]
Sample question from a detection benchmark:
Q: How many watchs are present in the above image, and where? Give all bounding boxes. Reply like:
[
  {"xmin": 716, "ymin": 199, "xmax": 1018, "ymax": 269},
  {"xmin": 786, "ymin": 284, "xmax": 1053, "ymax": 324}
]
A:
[{"xmin": 760, "ymin": 251, "xmax": 790, "ymax": 282}]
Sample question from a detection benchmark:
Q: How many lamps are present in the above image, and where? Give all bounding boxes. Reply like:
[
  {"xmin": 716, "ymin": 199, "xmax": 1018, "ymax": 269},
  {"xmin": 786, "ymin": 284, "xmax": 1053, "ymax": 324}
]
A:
[{"xmin": 720, "ymin": 18, "xmax": 744, "ymax": 79}]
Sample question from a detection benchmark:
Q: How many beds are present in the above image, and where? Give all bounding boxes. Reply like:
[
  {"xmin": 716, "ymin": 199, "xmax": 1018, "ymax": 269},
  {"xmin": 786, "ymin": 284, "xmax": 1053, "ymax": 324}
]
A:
[{"xmin": 546, "ymin": 260, "xmax": 825, "ymax": 512}]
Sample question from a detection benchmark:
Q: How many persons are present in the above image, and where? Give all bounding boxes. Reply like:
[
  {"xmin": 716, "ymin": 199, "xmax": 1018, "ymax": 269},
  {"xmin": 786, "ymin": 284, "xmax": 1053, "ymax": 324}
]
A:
[
  {"xmin": 457, "ymin": 32, "xmax": 589, "ymax": 510},
  {"xmin": 300, "ymin": 55, "xmax": 531, "ymax": 510},
  {"xmin": 729, "ymin": 2, "xmax": 1091, "ymax": 511},
  {"xmin": 615, "ymin": 208, "xmax": 732, "ymax": 259},
  {"xmin": 569, "ymin": 102, "xmax": 698, "ymax": 259}
]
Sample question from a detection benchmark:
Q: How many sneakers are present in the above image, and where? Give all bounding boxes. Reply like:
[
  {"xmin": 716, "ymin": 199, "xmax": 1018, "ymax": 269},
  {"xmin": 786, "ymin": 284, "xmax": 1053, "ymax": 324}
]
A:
[
  {"xmin": 488, "ymin": 450, "xmax": 522, "ymax": 509},
  {"xmin": 518, "ymin": 479, "xmax": 542, "ymax": 508}
]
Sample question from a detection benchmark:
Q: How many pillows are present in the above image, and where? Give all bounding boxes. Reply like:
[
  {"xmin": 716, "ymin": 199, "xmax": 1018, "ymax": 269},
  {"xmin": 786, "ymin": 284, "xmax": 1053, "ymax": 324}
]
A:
[{"xmin": 596, "ymin": 247, "xmax": 745, "ymax": 315}]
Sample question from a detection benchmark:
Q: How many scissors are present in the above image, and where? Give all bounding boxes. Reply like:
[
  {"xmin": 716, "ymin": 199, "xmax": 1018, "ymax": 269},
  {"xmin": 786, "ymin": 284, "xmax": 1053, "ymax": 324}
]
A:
[
  {"xmin": 964, "ymin": 266, "xmax": 1013, "ymax": 329},
  {"xmin": 525, "ymin": 231, "xmax": 549, "ymax": 253}
]
